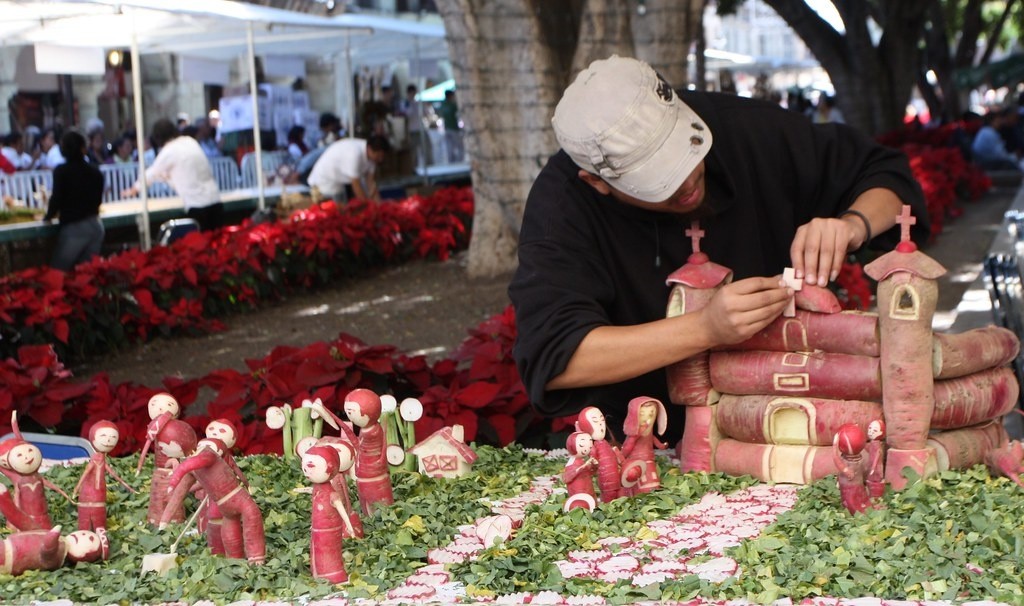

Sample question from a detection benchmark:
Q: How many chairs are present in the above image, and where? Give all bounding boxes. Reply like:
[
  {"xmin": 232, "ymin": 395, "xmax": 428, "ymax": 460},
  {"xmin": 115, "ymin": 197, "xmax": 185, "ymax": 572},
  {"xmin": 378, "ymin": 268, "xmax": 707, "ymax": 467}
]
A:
[{"xmin": 157, "ymin": 218, "xmax": 201, "ymax": 247}]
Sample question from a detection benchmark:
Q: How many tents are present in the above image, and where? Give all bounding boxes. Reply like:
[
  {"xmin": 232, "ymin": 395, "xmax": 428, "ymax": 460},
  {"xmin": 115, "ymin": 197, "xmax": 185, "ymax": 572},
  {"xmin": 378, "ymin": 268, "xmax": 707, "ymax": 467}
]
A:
[{"xmin": 0, "ymin": 0, "xmax": 455, "ymax": 245}]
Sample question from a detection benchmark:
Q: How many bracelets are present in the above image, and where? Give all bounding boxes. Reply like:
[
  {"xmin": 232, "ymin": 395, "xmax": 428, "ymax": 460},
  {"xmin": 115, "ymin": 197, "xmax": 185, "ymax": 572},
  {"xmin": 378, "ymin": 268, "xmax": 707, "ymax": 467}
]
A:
[{"xmin": 837, "ymin": 209, "xmax": 872, "ymax": 244}]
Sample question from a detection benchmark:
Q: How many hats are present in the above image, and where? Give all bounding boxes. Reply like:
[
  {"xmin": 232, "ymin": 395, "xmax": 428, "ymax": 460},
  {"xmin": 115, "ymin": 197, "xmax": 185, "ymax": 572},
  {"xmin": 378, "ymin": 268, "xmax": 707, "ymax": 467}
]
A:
[
  {"xmin": 176, "ymin": 112, "xmax": 188, "ymax": 121},
  {"xmin": 87, "ymin": 119, "xmax": 103, "ymax": 134},
  {"xmin": 551, "ymin": 54, "xmax": 713, "ymax": 203},
  {"xmin": 208, "ymin": 110, "xmax": 220, "ymax": 119},
  {"xmin": 26, "ymin": 126, "xmax": 39, "ymax": 134}
]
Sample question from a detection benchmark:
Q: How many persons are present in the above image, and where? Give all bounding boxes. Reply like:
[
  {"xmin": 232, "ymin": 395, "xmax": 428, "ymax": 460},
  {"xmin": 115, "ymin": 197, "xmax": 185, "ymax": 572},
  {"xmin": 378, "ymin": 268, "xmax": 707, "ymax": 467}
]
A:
[
  {"xmin": 765, "ymin": 88, "xmax": 846, "ymax": 125},
  {"xmin": 0, "ymin": 82, "xmax": 466, "ymax": 271},
  {"xmin": 0, "ymin": 409, "xmax": 108, "ymax": 577},
  {"xmin": 508, "ymin": 55, "xmax": 929, "ymax": 450},
  {"xmin": 970, "ymin": 89, "xmax": 1024, "ymax": 169},
  {"xmin": 832, "ymin": 418, "xmax": 887, "ymax": 512},
  {"xmin": 136, "ymin": 393, "xmax": 265, "ymax": 560},
  {"xmin": 562, "ymin": 396, "xmax": 667, "ymax": 516},
  {"xmin": 73, "ymin": 420, "xmax": 139, "ymax": 533},
  {"xmin": 293, "ymin": 389, "xmax": 394, "ymax": 585}
]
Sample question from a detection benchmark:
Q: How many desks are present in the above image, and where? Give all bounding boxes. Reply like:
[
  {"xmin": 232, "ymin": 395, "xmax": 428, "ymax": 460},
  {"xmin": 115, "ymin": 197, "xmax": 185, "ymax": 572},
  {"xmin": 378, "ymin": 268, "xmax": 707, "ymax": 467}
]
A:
[{"xmin": 0, "ymin": 163, "xmax": 473, "ymax": 274}]
